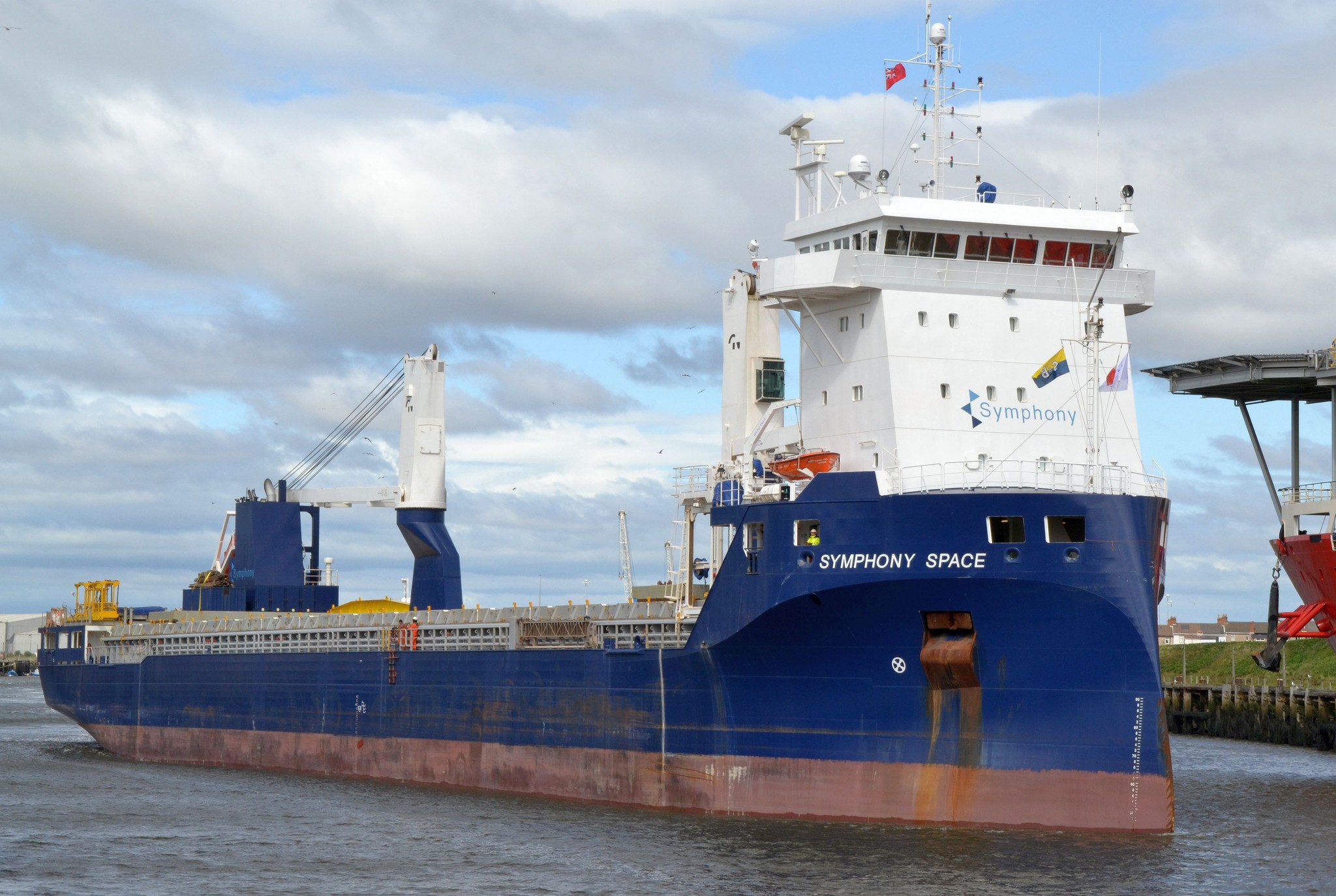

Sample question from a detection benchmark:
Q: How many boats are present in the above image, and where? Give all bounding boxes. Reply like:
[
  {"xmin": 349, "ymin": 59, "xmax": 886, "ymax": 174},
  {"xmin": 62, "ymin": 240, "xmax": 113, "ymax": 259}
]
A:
[
  {"xmin": 768, "ymin": 452, "xmax": 841, "ymax": 481},
  {"xmin": 8, "ymin": 670, "xmax": 18, "ymax": 676},
  {"xmin": 29, "ymin": 669, "xmax": 40, "ymax": 676},
  {"xmin": 37, "ymin": 0, "xmax": 1174, "ymax": 834}
]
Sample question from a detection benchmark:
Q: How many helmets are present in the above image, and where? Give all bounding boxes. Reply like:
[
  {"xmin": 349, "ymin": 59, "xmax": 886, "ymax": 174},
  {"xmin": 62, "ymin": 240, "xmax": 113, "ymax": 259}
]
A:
[{"xmin": 810, "ymin": 529, "xmax": 817, "ymax": 534}]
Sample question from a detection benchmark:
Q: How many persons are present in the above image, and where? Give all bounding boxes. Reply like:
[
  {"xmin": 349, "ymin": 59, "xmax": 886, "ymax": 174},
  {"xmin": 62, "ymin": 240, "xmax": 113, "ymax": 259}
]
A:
[
  {"xmin": 396, "ymin": 617, "xmax": 418, "ymax": 650},
  {"xmin": 807, "ymin": 529, "xmax": 820, "ymax": 546}
]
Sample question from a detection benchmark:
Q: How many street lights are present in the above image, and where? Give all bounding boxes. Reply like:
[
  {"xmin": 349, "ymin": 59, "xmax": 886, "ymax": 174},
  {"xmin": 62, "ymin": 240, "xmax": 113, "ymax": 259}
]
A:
[
  {"xmin": 582, "ymin": 579, "xmax": 589, "ymax": 605},
  {"xmin": 1164, "ymin": 594, "xmax": 1173, "ymax": 620}
]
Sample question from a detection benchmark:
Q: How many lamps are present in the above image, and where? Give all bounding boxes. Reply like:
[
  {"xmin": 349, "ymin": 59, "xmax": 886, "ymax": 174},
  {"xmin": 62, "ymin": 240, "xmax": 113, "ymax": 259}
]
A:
[
  {"xmin": 1099, "ymin": 297, "xmax": 1104, "ymax": 305},
  {"xmin": 1098, "ymin": 318, "xmax": 1104, "ymax": 327}
]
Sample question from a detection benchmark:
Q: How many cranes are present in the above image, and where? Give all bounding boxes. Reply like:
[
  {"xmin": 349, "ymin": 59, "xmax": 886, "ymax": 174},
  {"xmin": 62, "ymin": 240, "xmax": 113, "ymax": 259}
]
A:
[
  {"xmin": 402, "ymin": 578, "xmax": 408, "ymax": 603},
  {"xmin": 664, "ymin": 539, "xmax": 676, "ymax": 585},
  {"xmin": 617, "ymin": 510, "xmax": 633, "ymax": 605}
]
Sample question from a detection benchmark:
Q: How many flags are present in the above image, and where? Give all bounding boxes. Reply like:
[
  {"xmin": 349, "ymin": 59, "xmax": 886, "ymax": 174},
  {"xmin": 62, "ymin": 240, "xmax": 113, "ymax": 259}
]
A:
[
  {"xmin": 1098, "ymin": 352, "xmax": 1128, "ymax": 392},
  {"xmin": 885, "ymin": 63, "xmax": 906, "ymax": 91},
  {"xmin": 1032, "ymin": 347, "xmax": 1070, "ymax": 388}
]
